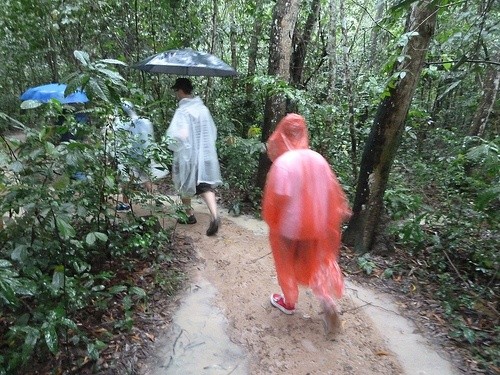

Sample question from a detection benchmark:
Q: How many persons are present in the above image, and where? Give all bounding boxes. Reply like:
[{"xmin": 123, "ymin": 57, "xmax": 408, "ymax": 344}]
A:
[
  {"xmin": 265, "ymin": 111, "xmax": 351, "ymax": 331},
  {"xmin": 44, "ymin": 100, "xmax": 170, "ymax": 214},
  {"xmin": 165, "ymin": 77, "xmax": 223, "ymax": 236}
]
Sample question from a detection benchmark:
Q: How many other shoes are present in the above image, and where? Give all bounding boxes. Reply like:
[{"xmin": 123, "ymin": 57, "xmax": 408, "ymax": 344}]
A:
[
  {"xmin": 177, "ymin": 214, "xmax": 197, "ymax": 225},
  {"xmin": 200, "ymin": 213, "xmax": 220, "ymax": 235},
  {"xmin": 114, "ymin": 203, "xmax": 130, "ymax": 212}
]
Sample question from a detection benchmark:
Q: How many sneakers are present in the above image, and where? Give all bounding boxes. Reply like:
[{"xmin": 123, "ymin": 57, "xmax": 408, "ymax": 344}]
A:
[{"xmin": 271, "ymin": 293, "xmax": 296, "ymax": 314}]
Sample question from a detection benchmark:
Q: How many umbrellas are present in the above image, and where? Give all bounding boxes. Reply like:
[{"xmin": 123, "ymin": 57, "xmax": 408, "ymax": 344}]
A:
[
  {"xmin": 127, "ymin": 44, "xmax": 238, "ymax": 110},
  {"xmin": 19, "ymin": 78, "xmax": 88, "ymax": 108}
]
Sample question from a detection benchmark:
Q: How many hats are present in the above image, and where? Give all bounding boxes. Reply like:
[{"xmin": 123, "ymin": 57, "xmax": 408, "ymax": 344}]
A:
[{"xmin": 170, "ymin": 77, "xmax": 191, "ymax": 90}]
